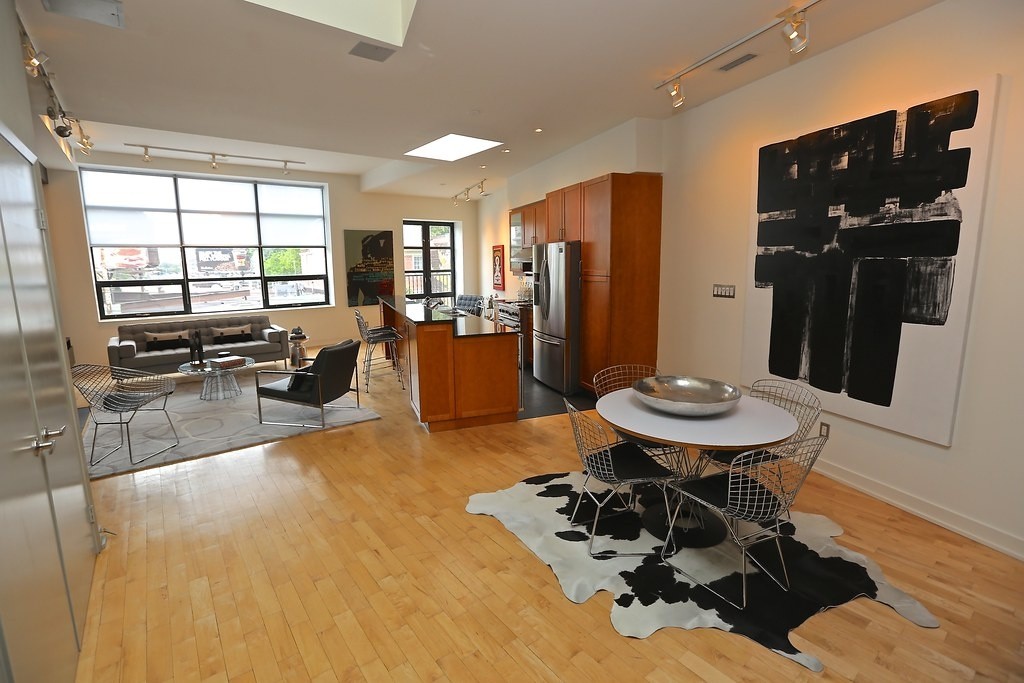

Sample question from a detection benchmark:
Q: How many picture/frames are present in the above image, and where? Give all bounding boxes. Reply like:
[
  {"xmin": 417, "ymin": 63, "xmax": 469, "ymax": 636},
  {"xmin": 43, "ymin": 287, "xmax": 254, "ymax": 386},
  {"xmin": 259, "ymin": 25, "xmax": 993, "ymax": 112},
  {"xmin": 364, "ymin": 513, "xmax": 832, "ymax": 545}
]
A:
[
  {"xmin": 509, "ymin": 172, "xmax": 663, "ymax": 396},
  {"xmin": 492, "ymin": 245, "xmax": 505, "ymax": 291}
]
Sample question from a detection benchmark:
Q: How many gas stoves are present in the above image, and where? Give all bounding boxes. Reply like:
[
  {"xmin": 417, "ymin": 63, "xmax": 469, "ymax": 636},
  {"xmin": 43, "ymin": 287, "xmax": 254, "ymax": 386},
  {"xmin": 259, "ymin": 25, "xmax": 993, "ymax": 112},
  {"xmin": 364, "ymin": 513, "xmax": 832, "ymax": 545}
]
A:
[{"xmin": 497, "ymin": 300, "xmax": 533, "ymax": 322}]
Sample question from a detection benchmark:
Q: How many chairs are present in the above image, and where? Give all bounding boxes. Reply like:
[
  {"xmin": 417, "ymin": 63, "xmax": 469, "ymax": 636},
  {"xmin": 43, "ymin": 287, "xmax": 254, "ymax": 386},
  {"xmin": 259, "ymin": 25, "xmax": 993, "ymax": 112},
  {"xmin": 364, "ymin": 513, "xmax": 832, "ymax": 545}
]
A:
[
  {"xmin": 455, "ymin": 295, "xmax": 485, "ymax": 316},
  {"xmin": 70, "ymin": 363, "xmax": 179, "ymax": 465},
  {"xmin": 563, "ymin": 362, "xmax": 827, "ymax": 610},
  {"xmin": 255, "ymin": 338, "xmax": 361, "ymax": 428}
]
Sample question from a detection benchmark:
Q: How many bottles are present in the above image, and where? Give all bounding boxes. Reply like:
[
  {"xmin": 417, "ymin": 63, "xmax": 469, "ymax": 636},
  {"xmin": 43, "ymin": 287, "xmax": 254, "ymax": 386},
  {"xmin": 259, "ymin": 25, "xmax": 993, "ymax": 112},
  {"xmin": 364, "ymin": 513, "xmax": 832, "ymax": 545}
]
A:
[
  {"xmin": 517, "ymin": 282, "xmax": 533, "ymax": 301},
  {"xmin": 495, "ymin": 294, "xmax": 498, "ymax": 298},
  {"xmin": 488, "ymin": 295, "xmax": 494, "ymax": 309}
]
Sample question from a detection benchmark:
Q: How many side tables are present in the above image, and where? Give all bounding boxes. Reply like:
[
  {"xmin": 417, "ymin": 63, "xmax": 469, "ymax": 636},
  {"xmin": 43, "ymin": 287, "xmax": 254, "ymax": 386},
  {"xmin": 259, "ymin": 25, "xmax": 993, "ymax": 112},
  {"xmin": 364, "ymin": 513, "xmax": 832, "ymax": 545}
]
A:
[{"xmin": 289, "ymin": 333, "xmax": 310, "ymax": 367}]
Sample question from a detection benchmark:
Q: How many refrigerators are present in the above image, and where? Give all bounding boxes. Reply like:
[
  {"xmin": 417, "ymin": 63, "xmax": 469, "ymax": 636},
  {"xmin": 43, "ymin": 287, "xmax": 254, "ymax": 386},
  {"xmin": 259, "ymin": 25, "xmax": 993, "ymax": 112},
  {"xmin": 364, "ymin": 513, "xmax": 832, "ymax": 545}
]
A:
[{"xmin": 531, "ymin": 241, "xmax": 580, "ymax": 397}]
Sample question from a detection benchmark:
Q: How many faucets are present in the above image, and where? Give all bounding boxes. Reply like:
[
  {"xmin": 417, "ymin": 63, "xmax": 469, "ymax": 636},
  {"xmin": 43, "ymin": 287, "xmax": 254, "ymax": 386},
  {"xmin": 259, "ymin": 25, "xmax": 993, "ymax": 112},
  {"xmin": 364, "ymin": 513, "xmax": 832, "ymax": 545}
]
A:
[
  {"xmin": 422, "ymin": 295, "xmax": 432, "ymax": 306},
  {"xmin": 431, "ymin": 300, "xmax": 445, "ymax": 309}
]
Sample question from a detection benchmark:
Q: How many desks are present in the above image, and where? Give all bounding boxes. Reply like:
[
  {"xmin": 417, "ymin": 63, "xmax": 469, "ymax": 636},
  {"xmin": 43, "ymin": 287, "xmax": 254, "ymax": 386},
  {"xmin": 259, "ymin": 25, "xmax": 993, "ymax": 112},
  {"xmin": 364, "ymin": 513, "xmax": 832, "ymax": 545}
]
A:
[
  {"xmin": 377, "ymin": 295, "xmax": 523, "ymax": 434},
  {"xmin": 178, "ymin": 356, "xmax": 255, "ymax": 401},
  {"xmin": 595, "ymin": 384, "xmax": 798, "ymax": 533}
]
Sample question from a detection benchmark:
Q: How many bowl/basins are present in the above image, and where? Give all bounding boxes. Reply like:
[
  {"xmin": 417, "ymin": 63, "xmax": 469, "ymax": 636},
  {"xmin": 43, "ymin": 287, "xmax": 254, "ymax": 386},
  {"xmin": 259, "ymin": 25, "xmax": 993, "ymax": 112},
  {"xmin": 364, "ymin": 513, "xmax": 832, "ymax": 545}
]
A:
[{"xmin": 632, "ymin": 374, "xmax": 743, "ymax": 416}]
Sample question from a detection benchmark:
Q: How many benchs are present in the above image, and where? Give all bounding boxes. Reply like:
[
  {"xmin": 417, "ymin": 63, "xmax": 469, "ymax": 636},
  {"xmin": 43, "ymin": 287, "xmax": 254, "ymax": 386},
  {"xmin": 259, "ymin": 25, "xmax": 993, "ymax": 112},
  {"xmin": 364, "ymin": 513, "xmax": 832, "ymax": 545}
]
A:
[{"xmin": 107, "ymin": 315, "xmax": 290, "ymax": 386}]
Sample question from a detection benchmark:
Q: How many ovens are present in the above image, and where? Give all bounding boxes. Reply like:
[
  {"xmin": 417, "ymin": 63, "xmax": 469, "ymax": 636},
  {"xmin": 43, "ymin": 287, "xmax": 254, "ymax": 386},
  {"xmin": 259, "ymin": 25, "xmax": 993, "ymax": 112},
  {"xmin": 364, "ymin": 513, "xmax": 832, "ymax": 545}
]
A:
[{"xmin": 499, "ymin": 317, "xmax": 522, "ymax": 368}]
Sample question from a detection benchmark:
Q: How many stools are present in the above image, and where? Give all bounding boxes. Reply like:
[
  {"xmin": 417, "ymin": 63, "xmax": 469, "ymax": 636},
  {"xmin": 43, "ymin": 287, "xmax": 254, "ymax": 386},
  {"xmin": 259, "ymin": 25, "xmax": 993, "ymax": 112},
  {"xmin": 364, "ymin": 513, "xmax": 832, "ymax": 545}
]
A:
[{"xmin": 355, "ymin": 309, "xmax": 405, "ymax": 393}]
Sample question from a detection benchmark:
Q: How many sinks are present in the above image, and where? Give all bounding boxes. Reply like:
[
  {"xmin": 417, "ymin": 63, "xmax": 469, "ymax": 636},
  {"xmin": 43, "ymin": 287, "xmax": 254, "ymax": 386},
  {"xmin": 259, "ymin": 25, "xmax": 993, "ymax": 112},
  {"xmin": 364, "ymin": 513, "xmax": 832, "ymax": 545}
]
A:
[
  {"xmin": 446, "ymin": 313, "xmax": 467, "ymax": 318},
  {"xmin": 440, "ymin": 311, "xmax": 460, "ymax": 314}
]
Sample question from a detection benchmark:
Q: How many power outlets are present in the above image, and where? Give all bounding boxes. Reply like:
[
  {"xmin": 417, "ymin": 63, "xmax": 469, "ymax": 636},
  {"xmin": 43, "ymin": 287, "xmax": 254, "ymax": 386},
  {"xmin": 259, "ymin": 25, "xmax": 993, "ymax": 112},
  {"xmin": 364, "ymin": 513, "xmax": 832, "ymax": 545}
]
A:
[
  {"xmin": 713, "ymin": 284, "xmax": 735, "ymax": 298},
  {"xmin": 820, "ymin": 422, "xmax": 830, "ymax": 439}
]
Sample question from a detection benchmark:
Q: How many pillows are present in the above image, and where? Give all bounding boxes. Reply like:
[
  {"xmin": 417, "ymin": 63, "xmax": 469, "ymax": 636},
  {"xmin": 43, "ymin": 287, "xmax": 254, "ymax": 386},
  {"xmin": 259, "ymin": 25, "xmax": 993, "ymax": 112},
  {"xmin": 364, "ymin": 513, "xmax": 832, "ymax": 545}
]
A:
[
  {"xmin": 143, "ymin": 323, "xmax": 255, "ymax": 352},
  {"xmin": 287, "ymin": 365, "xmax": 311, "ymax": 391}
]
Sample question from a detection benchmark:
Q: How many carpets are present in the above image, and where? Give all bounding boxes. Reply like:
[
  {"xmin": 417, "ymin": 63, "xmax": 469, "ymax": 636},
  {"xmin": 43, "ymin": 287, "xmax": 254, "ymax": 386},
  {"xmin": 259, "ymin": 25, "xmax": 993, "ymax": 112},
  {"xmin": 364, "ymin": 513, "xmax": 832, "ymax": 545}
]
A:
[
  {"xmin": 465, "ymin": 448, "xmax": 939, "ymax": 672},
  {"xmin": 83, "ymin": 362, "xmax": 381, "ymax": 481}
]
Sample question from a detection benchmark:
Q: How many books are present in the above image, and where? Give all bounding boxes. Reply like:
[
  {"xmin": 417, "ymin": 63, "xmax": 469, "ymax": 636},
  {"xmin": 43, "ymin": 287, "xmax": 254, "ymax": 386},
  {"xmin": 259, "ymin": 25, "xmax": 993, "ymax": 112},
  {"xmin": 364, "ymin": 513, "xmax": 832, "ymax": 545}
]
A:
[{"xmin": 210, "ymin": 356, "xmax": 246, "ymax": 367}]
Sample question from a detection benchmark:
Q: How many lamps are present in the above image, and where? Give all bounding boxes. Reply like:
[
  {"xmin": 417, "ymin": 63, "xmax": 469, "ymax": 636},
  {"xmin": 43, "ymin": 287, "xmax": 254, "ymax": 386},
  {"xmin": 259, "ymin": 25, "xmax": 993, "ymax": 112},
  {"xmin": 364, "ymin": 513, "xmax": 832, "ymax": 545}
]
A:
[
  {"xmin": 451, "ymin": 12, "xmax": 830, "ymax": 207},
  {"xmin": 18, "ymin": 25, "xmax": 291, "ymax": 177}
]
